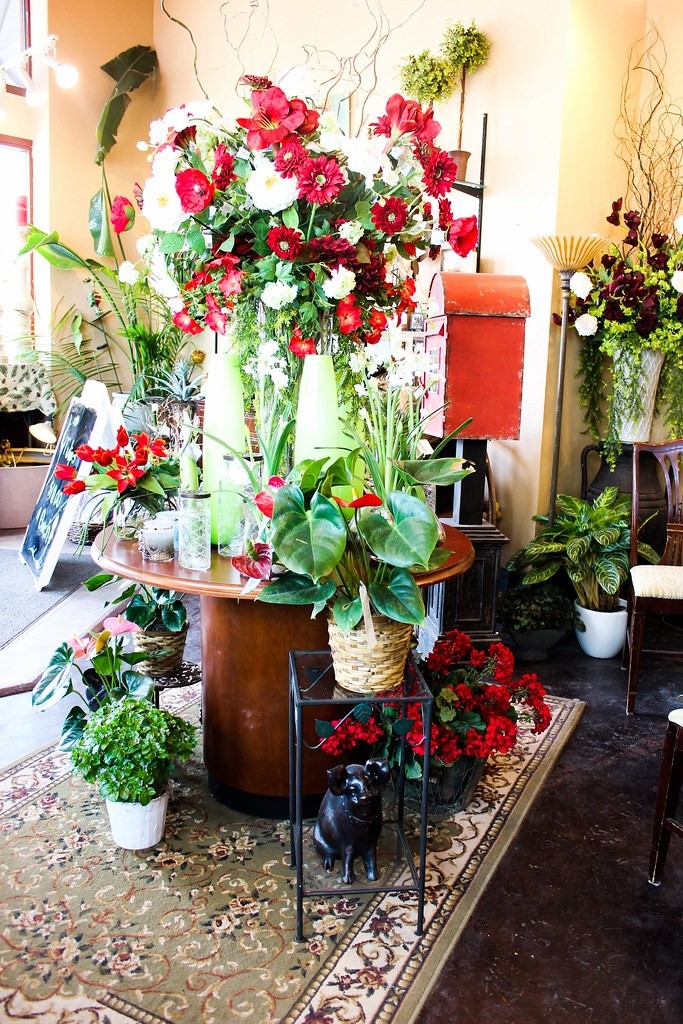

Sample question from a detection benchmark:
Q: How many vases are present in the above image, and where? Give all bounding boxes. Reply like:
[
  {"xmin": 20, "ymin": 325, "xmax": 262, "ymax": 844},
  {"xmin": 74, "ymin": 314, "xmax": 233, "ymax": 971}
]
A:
[
  {"xmin": 324, "ymin": 610, "xmax": 411, "ymax": 691},
  {"xmin": 113, "ymin": 391, "xmax": 133, "ymax": 416},
  {"xmin": 394, "ymin": 707, "xmax": 516, "ymax": 802},
  {"xmin": 295, "ymin": 355, "xmax": 346, "ymax": 474},
  {"xmin": 202, "ymin": 333, "xmax": 254, "ymax": 546},
  {"xmin": 610, "ymin": 346, "xmax": 665, "ymax": 443}
]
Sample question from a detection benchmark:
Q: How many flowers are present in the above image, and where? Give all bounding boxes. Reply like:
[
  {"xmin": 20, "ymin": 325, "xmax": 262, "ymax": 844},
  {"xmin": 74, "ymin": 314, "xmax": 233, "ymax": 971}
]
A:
[
  {"xmin": 19, "ymin": 18, "xmax": 487, "ymax": 628},
  {"xmin": 552, "ymin": 196, "xmax": 683, "ymax": 465},
  {"xmin": 31, "ymin": 617, "xmax": 173, "ymax": 758},
  {"xmin": 310, "ymin": 629, "xmax": 555, "ymax": 778}
]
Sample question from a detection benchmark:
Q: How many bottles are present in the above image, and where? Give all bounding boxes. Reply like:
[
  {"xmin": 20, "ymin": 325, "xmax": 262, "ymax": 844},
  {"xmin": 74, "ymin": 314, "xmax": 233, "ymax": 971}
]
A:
[
  {"xmin": 218, "ymin": 452, "xmax": 263, "ymax": 557},
  {"xmin": 179, "ymin": 490, "xmax": 211, "ymax": 571}
]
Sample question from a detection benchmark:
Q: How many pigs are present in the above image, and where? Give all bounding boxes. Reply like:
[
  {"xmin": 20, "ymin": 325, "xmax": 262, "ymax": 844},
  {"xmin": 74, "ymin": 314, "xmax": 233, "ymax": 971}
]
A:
[{"xmin": 313, "ymin": 759, "xmax": 391, "ymax": 885}]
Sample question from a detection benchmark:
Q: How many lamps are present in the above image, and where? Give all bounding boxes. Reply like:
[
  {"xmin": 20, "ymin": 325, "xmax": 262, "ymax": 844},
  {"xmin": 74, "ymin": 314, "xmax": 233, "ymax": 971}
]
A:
[{"xmin": 22, "ymin": 33, "xmax": 79, "ymax": 91}]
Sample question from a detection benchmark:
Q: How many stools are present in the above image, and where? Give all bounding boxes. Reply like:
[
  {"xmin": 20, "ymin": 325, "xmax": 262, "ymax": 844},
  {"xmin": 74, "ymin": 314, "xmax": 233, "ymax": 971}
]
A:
[{"xmin": 287, "ymin": 648, "xmax": 434, "ymax": 943}]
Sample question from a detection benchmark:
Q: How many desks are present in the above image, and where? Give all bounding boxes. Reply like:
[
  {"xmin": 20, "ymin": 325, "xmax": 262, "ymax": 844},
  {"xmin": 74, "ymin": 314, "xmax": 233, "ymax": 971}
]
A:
[{"xmin": 93, "ymin": 515, "xmax": 474, "ymax": 820}]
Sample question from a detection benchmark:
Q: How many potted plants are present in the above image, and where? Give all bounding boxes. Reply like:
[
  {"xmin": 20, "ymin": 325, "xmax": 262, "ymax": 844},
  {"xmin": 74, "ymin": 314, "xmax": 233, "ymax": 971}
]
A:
[
  {"xmin": 505, "ymin": 489, "xmax": 662, "ymax": 655},
  {"xmin": 73, "ymin": 693, "xmax": 200, "ymax": 850},
  {"xmin": 85, "ymin": 565, "xmax": 191, "ymax": 673}
]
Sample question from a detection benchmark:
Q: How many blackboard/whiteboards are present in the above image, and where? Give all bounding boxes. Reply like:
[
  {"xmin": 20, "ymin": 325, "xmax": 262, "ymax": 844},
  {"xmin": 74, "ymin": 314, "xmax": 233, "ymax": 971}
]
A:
[{"xmin": 19, "ymin": 396, "xmax": 104, "ymax": 592}]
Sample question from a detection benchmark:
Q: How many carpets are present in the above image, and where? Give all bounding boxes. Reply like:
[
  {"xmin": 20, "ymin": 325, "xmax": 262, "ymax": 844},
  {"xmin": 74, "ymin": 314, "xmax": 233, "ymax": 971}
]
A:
[{"xmin": 0, "ymin": 660, "xmax": 588, "ymax": 1024}]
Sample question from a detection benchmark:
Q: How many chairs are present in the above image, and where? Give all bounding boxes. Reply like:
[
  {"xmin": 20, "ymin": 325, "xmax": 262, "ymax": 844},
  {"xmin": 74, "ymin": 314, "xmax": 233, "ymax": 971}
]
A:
[
  {"xmin": 619, "ymin": 440, "xmax": 683, "ymax": 714},
  {"xmin": 648, "ymin": 708, "xmax": 683, "ymax": 887}
]
